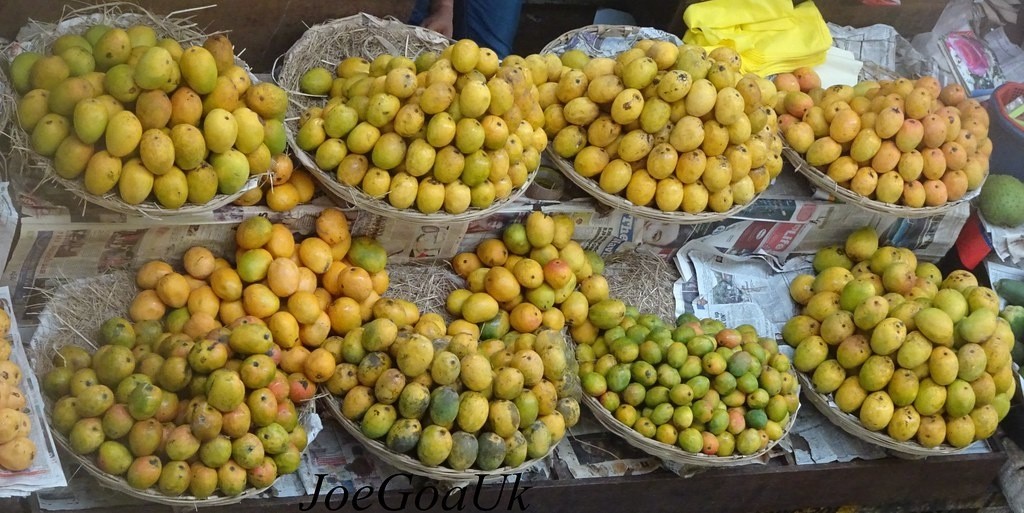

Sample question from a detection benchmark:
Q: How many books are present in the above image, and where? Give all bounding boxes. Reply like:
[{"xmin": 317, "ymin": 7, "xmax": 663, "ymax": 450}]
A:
[{"xmin": 938, "ymin": 29, "xmax": 1010, "ymax": 98}]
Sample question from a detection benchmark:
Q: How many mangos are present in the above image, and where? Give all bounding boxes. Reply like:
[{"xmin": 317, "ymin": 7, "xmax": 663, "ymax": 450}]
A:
[{"xmin": 0, "ymin": 26, "xmax": 1016, "ymax": 495}]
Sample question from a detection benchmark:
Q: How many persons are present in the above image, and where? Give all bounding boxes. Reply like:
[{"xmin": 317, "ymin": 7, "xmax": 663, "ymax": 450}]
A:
[{"xmin": 416, "ymin": 0, "xmax": 682, "ymax": 59}]
